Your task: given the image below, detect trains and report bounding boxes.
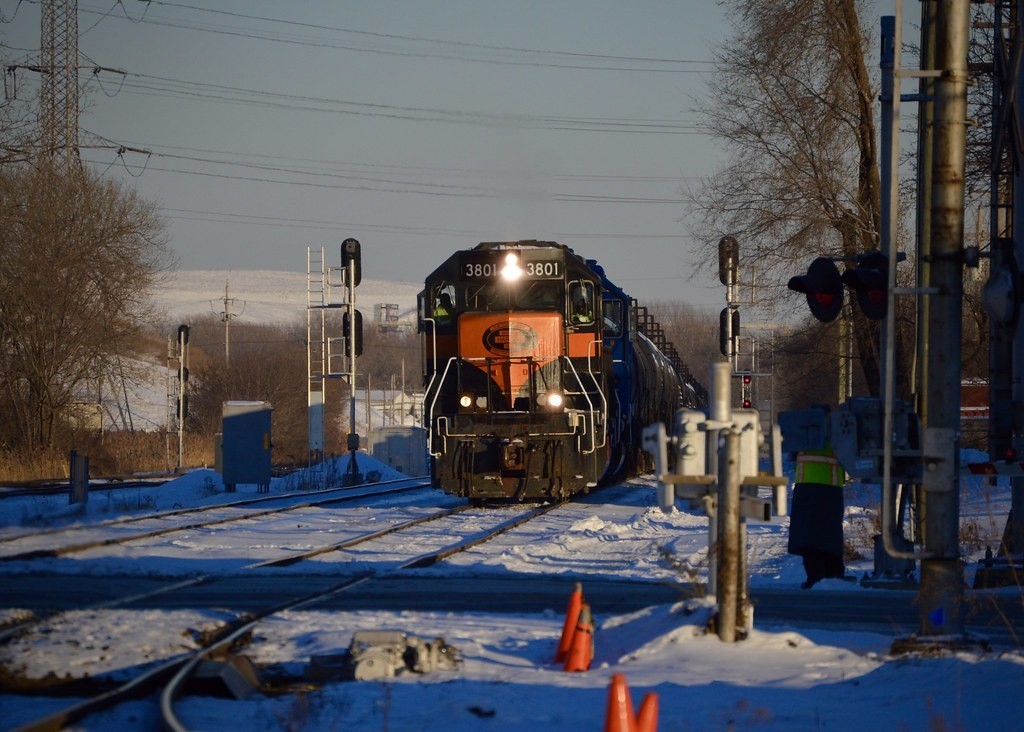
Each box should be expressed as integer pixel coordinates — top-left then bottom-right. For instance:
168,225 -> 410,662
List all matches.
417,239 -> 710,508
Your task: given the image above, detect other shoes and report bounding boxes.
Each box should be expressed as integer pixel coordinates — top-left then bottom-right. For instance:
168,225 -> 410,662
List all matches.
801,580 -> 816,589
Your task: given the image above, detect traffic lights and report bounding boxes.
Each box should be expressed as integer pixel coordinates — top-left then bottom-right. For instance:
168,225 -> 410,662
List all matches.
788,257 -> 844,323
842,250 -> 888,321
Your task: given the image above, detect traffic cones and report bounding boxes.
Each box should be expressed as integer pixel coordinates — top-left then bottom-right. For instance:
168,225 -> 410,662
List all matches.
562,603 -> 595,674
636,692 -> 659,732
602,672 -> 640,732
551,581 -> 584,665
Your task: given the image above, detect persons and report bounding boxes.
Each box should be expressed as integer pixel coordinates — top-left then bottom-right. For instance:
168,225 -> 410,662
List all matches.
434,294 -> 454,316
572,299 -> 592,322
790,404 -> 844,589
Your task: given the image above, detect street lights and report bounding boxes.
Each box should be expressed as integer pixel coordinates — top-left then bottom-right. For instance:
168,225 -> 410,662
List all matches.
744,368 -> 751,383
744,399 -> 751,408
